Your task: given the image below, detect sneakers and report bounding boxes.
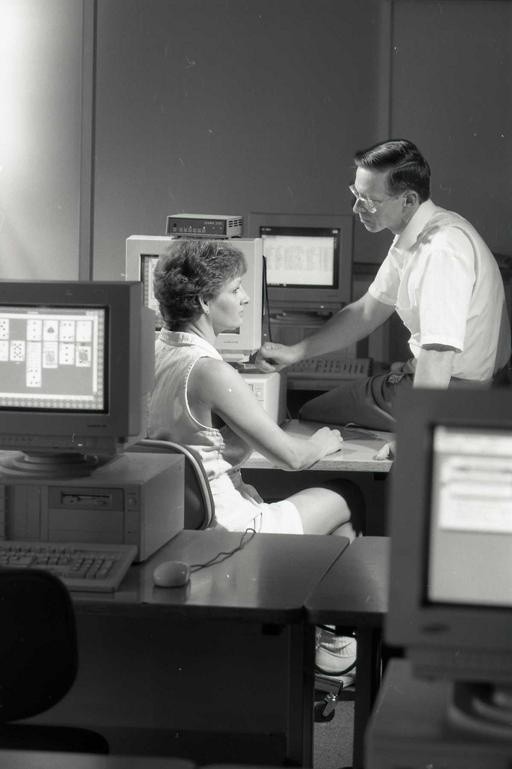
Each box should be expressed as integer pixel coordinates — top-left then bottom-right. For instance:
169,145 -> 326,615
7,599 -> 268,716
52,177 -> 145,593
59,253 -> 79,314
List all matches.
312,623 -> 356,675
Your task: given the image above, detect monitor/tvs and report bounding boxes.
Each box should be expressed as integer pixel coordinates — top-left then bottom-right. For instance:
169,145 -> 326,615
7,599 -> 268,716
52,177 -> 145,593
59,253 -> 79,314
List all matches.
246,212 -> 356,324
125,235 -> 265,362
383,387 -> 512,739
0,280 -> 156,477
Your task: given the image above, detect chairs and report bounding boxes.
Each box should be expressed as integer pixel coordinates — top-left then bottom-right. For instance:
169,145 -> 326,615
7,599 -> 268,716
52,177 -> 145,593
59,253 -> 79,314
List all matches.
0,568 -> 108,754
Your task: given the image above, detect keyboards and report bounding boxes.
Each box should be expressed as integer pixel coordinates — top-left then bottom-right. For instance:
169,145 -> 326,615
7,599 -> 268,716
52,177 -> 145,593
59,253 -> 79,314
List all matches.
0,540 -> 139,594
284,358 -> 373,380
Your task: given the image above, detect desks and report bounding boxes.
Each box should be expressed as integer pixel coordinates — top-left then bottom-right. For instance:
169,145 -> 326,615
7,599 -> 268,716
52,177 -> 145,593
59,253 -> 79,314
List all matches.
233,420 -> 391,538
306,535 -> 391,766
72,529 -> 352,767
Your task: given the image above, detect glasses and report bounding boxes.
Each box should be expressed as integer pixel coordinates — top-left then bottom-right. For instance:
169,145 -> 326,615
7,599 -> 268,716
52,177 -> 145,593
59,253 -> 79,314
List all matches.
349,180 -> 402,213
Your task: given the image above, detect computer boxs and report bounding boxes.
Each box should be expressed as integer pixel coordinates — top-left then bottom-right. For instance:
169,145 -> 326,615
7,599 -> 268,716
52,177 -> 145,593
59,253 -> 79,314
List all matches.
0,450 -> 185,563
234,362 -> 287,429
263,323 -> 368,359
361,656 -> 512,769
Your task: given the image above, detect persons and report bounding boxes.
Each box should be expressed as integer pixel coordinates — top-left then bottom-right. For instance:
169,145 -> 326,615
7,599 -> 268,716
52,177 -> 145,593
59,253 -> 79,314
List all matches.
137,236 -> 362,678
251,136 -> 512,534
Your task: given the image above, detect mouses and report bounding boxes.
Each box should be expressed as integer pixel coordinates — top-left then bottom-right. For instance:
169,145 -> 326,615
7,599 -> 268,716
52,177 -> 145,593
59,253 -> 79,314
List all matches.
390,361 -> 405,371
153,560 -> 190,586
